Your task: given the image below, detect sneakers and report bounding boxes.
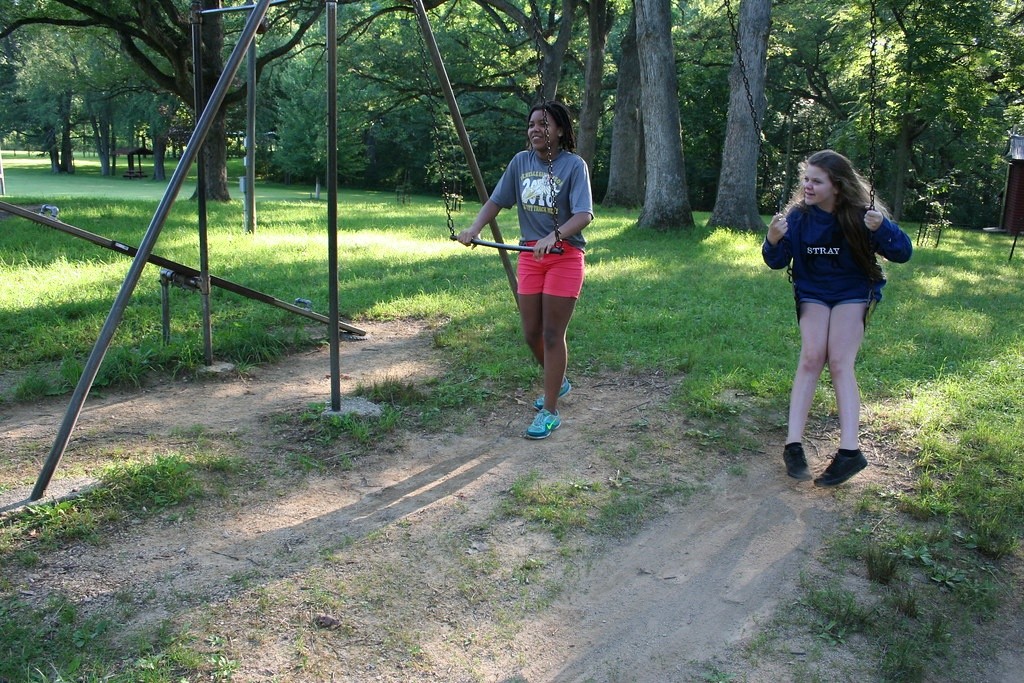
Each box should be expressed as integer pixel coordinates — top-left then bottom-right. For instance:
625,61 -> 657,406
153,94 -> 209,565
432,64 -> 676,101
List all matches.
524,407 -> 561,439
783,445 -> 812,481
533,375 -> 571,411
814,449 -> 867,488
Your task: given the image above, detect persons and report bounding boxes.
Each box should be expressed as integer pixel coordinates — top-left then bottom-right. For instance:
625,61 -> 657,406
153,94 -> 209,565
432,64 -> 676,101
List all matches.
456,100 -> 594,439
762,149 -> 914,486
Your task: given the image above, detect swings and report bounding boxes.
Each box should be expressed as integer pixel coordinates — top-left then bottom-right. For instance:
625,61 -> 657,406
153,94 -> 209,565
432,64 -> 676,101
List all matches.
416,0 -> 564,255
725,2 -> 882,333
